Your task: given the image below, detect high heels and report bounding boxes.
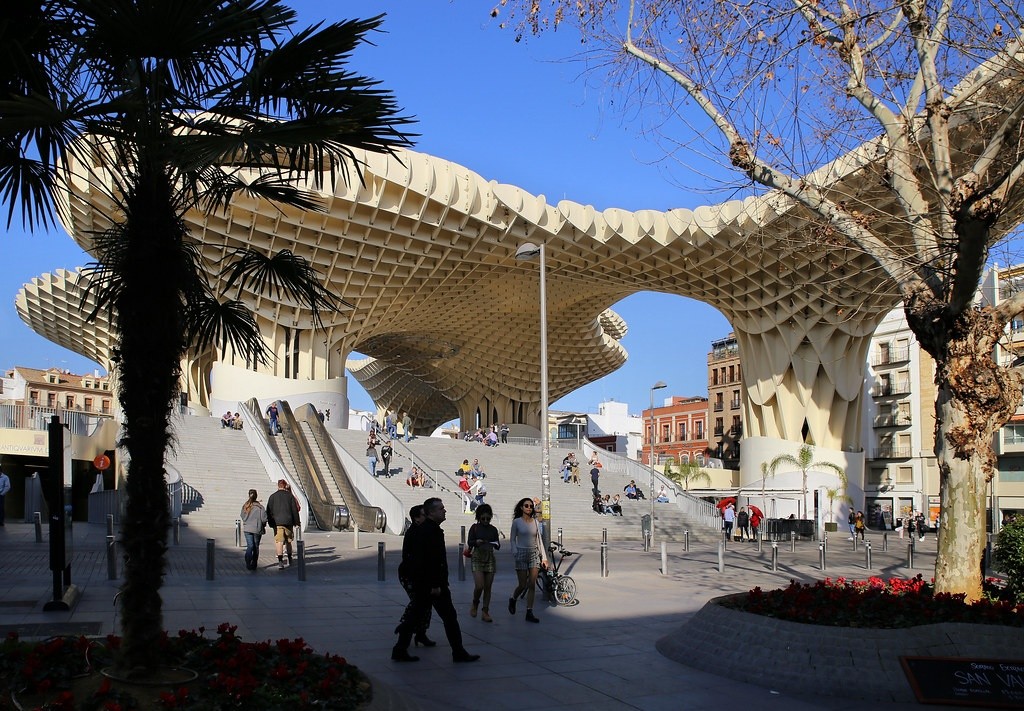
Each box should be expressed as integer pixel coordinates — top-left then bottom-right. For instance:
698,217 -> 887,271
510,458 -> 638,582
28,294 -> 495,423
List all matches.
414,635 -> 436,647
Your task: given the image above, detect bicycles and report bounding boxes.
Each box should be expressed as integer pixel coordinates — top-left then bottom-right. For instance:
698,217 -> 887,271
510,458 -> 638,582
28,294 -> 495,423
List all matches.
520,540 -> 578,606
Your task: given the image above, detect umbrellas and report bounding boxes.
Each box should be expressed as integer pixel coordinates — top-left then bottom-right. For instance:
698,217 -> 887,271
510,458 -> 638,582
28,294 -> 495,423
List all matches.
716,497 -> 736,509
746,505 -> 764,518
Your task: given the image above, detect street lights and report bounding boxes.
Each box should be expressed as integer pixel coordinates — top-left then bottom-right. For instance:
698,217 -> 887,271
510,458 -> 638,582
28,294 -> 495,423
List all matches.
515,242 -> 553,572
650,382 -> 668,548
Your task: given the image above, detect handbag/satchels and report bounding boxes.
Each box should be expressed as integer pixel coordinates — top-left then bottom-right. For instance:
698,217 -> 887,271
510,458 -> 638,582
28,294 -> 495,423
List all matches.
536,532 -> 547,561
595,462 -> 602,467
464,549 -> 473,558
383,451 -> 390,459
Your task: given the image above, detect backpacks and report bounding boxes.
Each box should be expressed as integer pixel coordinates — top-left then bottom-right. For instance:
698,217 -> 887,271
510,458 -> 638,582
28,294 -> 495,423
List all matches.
478,484 -> 487,496
455,468 -> 464,476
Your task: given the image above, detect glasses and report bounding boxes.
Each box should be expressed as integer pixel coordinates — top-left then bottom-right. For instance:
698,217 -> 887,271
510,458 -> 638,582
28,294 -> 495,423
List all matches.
524,504 -> 534,508
481,517 -> 491,521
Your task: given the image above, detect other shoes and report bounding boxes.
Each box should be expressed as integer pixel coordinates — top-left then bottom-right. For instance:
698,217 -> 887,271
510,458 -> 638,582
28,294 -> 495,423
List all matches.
525,609 -> 540,623
481,608 -> 493,622
278,561 -> 285,570
471,599 -> 480,617
288,557 -> 293,564
391,646 -> 421,662
452,649 -> 480,663
508,597 -> 517,615
246,561 -> 258,571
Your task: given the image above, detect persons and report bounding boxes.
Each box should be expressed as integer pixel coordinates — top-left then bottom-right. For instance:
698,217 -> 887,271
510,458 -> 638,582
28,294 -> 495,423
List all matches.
721,501 -> 760,542
390,497 -> 482,663
460,458 -> 484,480
276,485 -> 301,558
394,504 -> 438,648
221,411 -> 243,430
468,503 -> 501,622
847,507 -> 865,543
386,410 -> 412,443
407,467 -> 425,490
380,441 -> 393,478
559,450 -> 669,516
0,464 -> 10,526
240,489 -> 268,570
266,402 -> 279,436
934,512 -> 940,540
458,473 -> 487,513
366,442 -> 380,476
267,480 -> 300,569
508,498 -> 548,623
534,497 -> 543,535
318,409 -> 325,424
464,422 -> 510,447
367,430 -> 376,445
906,510 -> 926,542
